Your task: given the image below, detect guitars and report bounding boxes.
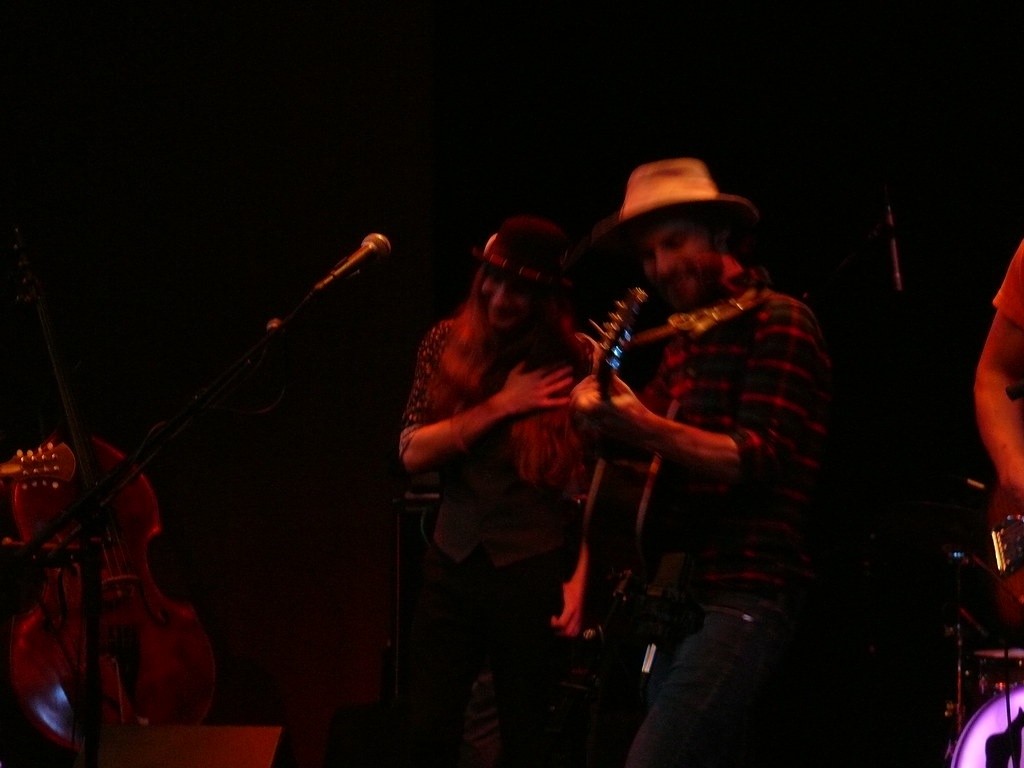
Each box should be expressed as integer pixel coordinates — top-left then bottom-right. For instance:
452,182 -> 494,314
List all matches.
535,279 -> 659,652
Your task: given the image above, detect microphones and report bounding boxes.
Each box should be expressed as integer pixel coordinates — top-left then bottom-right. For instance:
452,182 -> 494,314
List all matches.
889,206 -> 902,293
313,233 -> 392,291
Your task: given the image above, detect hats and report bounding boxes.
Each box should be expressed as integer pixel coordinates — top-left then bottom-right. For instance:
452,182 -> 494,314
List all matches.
593,158 -> 763,243
473,215 -> 577,291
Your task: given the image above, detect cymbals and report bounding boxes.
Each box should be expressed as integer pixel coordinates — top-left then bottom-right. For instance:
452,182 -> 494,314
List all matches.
974,644 -> 1024,660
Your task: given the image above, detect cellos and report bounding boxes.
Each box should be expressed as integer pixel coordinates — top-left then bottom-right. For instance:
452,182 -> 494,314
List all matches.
0,207 -> 227,761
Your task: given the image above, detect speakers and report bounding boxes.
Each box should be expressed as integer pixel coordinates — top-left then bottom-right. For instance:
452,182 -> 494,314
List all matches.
393,503 -> 503,768
74,723 -> 298,768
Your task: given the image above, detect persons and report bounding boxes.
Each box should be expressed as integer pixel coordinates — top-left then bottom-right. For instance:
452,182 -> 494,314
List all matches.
571,158 -> 833,768
972,238 -> 1024,627
396,217 -> 607,768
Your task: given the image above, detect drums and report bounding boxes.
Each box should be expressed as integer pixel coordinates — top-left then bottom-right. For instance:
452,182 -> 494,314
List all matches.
936,669 -> 1024,768
